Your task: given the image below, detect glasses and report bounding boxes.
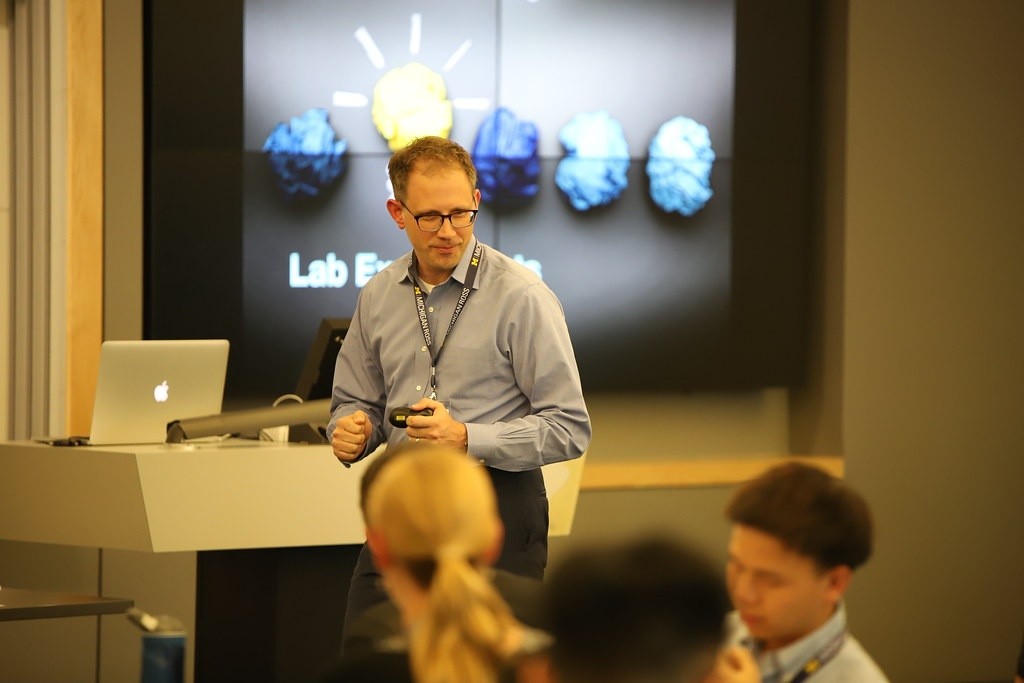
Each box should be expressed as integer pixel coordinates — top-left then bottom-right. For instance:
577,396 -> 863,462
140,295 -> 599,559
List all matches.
400,196 -> 478,234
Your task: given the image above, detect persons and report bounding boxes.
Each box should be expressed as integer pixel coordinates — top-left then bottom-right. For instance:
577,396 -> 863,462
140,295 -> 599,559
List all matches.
540,531 -> 737,683
314,440 -> 560,683
715,462 -> 892,683
324,136 -> 593,657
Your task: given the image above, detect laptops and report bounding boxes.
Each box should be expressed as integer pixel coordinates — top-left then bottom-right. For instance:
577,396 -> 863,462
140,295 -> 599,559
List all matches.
34,339 -> 231,446
